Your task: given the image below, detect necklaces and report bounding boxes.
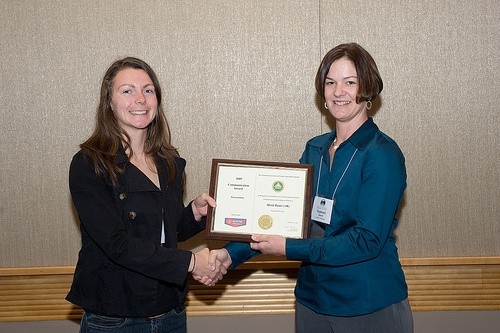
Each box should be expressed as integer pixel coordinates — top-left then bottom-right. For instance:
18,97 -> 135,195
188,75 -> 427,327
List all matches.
331,136 -> 350,150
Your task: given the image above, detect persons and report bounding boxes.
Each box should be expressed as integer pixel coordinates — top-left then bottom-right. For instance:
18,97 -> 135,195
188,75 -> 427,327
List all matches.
65,56 -> 228,333
192,41 -> 414,333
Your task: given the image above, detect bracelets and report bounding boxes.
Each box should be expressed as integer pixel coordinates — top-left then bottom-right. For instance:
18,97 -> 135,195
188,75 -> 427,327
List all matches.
190,251 -> 198,273
